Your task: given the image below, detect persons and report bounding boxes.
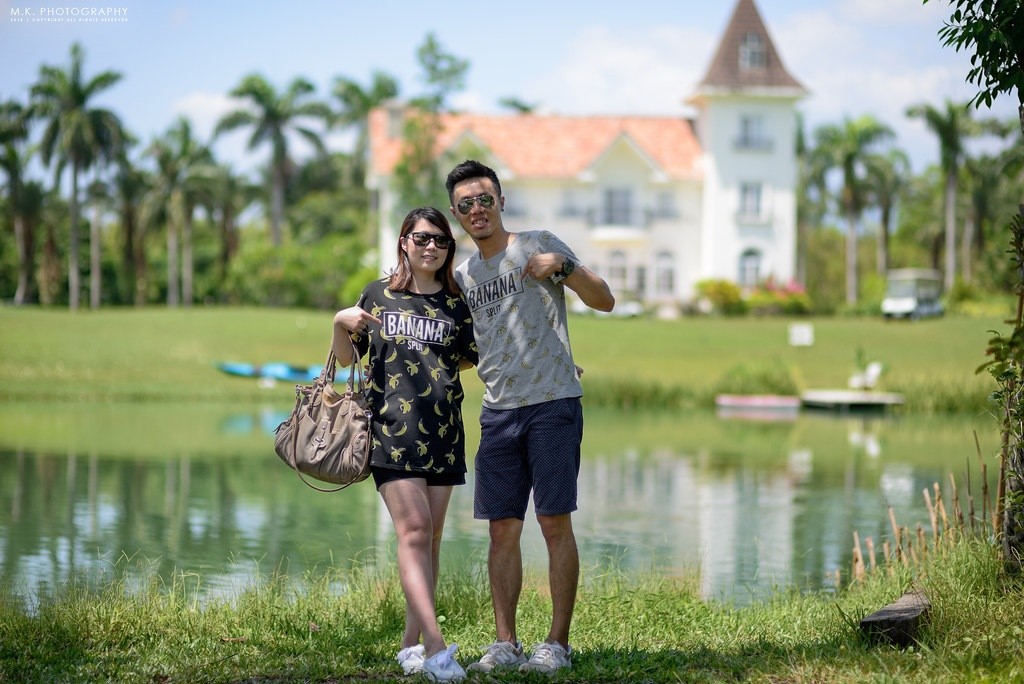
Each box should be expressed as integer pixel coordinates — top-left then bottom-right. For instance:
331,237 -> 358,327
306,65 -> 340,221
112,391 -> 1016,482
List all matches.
332,207 -> 583,684
364,160 -> 615,672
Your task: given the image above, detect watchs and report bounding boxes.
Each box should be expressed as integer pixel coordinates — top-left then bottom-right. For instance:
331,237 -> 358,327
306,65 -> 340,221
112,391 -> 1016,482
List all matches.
555,256 -> 575,280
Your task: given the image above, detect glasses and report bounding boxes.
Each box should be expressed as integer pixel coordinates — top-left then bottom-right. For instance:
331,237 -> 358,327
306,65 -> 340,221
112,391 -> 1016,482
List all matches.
457,194 -> 496,215
406,231 -> 452,249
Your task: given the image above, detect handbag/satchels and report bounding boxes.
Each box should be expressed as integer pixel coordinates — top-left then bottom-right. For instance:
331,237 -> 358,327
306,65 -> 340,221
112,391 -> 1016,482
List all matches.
272,341 -> 372,492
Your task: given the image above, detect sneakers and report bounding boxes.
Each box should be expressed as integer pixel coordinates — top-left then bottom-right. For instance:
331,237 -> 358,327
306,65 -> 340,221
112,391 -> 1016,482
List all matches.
397,643 -> 424,675
423,643 -> 466,683
466,641 -> 528,674
519,638 -> 572,674
